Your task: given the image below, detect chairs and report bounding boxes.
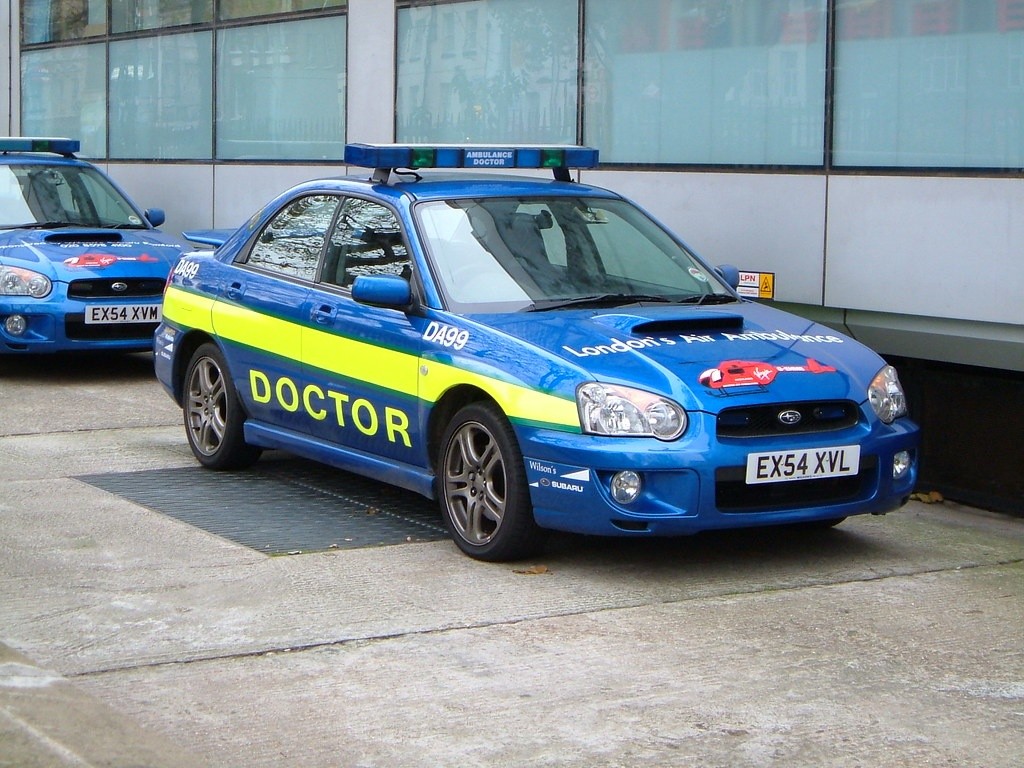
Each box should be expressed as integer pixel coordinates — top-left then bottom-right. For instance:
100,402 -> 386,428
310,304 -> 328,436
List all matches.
28,173 -> 69,224
497,213 -> 560,294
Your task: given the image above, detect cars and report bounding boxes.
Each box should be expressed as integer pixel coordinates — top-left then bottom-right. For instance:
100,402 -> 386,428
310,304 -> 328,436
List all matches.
0,136 -> 186,361
151,140 -> 921,562
